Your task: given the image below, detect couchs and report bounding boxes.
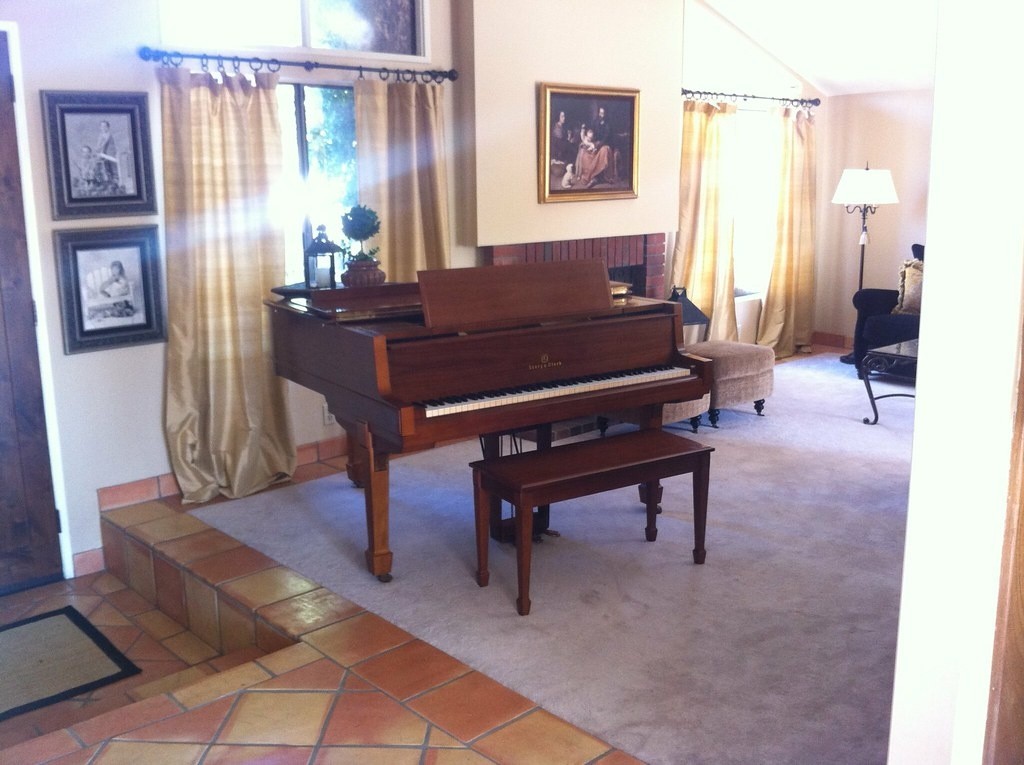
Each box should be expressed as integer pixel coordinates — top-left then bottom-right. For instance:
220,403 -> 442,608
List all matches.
852,244 -> 924,366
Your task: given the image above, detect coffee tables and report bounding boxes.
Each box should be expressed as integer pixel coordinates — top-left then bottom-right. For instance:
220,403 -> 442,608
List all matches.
860,336 -> 920,426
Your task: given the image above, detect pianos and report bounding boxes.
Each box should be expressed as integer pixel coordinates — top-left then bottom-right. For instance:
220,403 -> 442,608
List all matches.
263,257 -> 715,582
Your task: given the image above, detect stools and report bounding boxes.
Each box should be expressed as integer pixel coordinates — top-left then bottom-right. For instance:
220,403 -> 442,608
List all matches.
598,340 -> 779,435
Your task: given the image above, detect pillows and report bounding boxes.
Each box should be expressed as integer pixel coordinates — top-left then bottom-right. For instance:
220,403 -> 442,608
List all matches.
893,258 -> 924,315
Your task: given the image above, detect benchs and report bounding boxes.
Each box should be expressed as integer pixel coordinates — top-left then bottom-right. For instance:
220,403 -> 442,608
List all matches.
470,431 -> 714,617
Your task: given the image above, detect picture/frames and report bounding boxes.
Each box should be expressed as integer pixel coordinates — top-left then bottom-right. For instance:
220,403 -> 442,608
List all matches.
340,205 -> 387,288
536,82 -> 643,204
52,224 -> 169,356
38,90 -> 160,220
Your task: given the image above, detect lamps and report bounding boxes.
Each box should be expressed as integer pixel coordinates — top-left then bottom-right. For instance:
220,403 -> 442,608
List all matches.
831,159 -> 899,363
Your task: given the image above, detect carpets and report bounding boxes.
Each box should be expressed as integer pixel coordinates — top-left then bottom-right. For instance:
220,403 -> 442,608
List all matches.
0,605 -> 143,725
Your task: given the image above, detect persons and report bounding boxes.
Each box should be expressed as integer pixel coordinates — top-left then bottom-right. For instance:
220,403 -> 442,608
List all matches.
552,106 -> 615,190
81,121 -> 119,188
99,261 -> 134,317
